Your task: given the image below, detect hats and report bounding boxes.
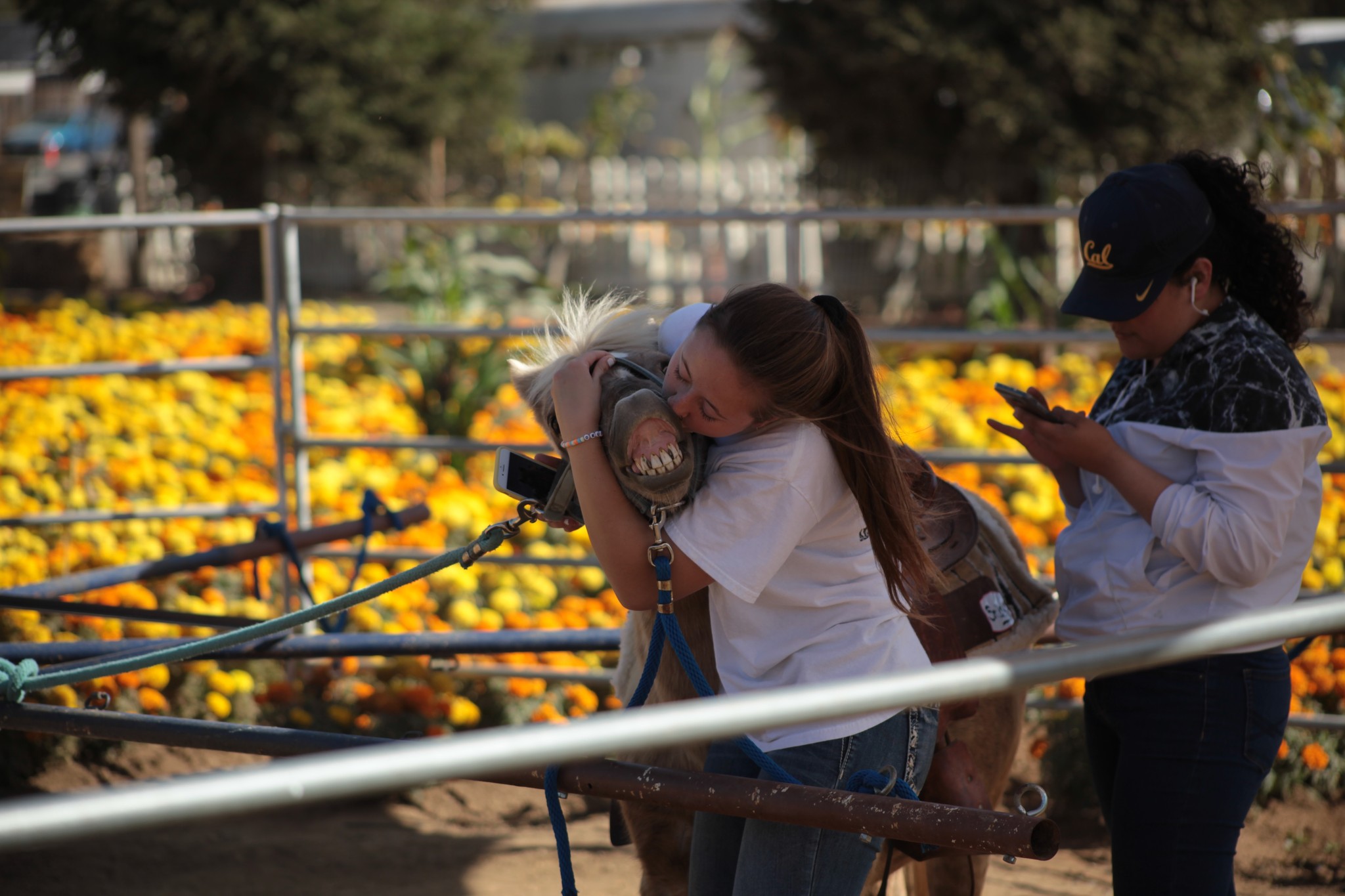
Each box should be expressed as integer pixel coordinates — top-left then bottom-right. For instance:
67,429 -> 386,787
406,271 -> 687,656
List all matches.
1056,167 -> 1216,320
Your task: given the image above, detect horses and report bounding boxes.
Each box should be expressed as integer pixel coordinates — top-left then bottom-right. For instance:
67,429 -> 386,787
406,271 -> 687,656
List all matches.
507,283 -> 1055,895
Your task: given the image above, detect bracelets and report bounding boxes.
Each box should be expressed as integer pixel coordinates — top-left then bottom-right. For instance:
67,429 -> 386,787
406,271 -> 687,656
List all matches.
561,431 -> 603,448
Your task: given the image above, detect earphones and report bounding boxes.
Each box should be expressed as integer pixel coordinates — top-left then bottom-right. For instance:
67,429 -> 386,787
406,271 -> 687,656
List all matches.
1190,277 -> 1198,305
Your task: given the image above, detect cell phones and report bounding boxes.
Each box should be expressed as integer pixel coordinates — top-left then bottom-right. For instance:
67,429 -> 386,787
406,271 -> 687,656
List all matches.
994,382 -> 1063,424
493,446 -> 570,518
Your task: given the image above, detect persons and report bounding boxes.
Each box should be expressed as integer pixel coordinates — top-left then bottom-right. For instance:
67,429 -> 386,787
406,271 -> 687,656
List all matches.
533,283 -> 941,896
985,146 -> 1331,896
